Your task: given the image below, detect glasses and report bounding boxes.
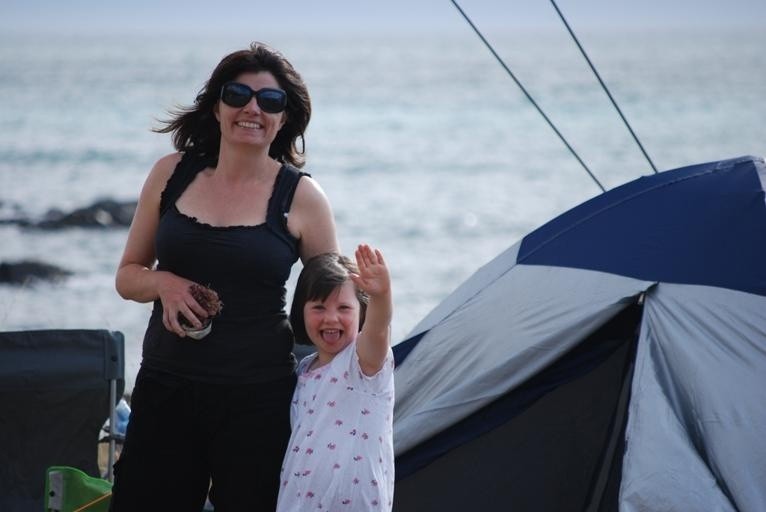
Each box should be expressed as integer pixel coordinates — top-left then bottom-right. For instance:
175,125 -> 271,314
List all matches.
220,80 -> 289,114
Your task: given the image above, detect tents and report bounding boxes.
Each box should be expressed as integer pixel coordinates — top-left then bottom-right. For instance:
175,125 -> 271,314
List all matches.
395,155 -> 766,512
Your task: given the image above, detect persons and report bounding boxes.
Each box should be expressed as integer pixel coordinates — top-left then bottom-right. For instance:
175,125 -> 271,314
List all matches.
108,39 -> 342,512
275,244 -> 395,512
